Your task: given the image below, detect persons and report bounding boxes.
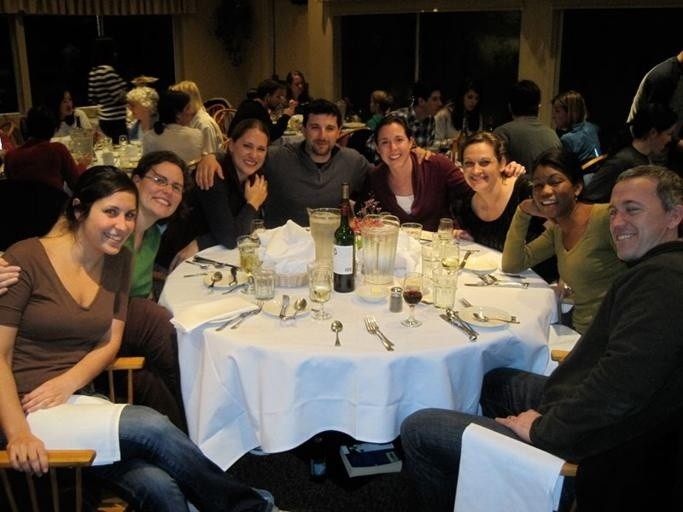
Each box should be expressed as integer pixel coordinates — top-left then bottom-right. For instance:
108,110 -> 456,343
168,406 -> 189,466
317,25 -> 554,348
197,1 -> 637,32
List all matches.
157,114 -> 270,273
0,165 -> 271,508
228,68 -> 312,143
502,146 -> 630,336
351,115 -> 527,248
0,149 -> 191,297
400,164 -> 683,508
452,131 -> 559,285
195,98 -> 436,227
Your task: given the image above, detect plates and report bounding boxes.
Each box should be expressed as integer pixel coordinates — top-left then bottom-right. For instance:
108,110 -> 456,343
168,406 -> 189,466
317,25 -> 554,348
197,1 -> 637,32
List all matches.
208,312 -> 240,324
456,254 -> 498,272
342,123 -> 365,128
201,269 -> 248,288
459,304 -> 512,329
262,297 -> 310,319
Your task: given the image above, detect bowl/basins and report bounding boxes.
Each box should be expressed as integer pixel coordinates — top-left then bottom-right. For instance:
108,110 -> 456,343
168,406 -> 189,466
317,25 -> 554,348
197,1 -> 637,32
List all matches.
352,285 -> 387,303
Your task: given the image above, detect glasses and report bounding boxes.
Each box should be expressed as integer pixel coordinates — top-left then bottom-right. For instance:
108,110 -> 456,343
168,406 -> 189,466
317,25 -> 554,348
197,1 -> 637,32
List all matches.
143,175 -> 185,195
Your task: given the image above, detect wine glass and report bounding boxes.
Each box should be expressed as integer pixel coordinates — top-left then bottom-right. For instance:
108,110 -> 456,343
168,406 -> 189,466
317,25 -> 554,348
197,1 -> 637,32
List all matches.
306,263 -> 337,320
401,271 -> 426,329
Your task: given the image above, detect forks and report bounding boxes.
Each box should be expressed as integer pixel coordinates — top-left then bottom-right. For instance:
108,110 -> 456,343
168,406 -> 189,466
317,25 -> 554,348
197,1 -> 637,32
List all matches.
459,298 -> 471,307
215,300 -> 267,333
363,313 -> 395,352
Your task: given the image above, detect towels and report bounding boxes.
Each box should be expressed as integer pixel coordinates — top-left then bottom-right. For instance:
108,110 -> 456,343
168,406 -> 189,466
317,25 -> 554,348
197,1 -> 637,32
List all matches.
452,423 -> 568,512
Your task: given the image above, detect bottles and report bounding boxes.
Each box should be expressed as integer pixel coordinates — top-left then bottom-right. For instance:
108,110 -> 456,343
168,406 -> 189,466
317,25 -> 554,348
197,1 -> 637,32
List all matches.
339,182 -> 355,230
389,287 -> 403,313
334,205 -> 355,293
451,138 -> 459,166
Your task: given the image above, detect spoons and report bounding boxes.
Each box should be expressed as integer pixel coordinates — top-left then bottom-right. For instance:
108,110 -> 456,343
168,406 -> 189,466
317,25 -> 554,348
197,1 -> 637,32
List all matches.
330,319 -> 344,348
464,273 -> 530,289
208,269 -> 222,288
183,257 -> 235,269
469,309 -> 523,325
288,296 -> 306,321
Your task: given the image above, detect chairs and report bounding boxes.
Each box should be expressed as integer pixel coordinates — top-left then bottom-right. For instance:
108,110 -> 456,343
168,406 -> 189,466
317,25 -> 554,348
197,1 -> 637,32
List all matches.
0,355 -> 145,512
455,393 -> 683,512
580,153 -> 609,173
336,127 -> 372,152
203,98 -> 238,133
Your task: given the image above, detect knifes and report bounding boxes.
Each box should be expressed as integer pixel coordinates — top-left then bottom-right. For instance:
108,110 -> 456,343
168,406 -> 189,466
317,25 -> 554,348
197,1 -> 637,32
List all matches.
221,282 -> 248,295
438,308 -> 477,340
227,266 -> 237,286
460,250 -> 473,269
279,294 -> 291,317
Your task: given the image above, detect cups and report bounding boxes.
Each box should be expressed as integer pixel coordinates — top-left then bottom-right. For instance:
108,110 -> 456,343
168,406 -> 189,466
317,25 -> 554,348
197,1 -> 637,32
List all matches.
359,212 -> 400,284
309,207 -> 341,264
235,234 -> 261,269
68,127 -> 93,162
400,217 -> 461,313
351,116 -> 358,123
101,135 -> 138,168
250,218 -> 264,236
252,262 -> 276,300
